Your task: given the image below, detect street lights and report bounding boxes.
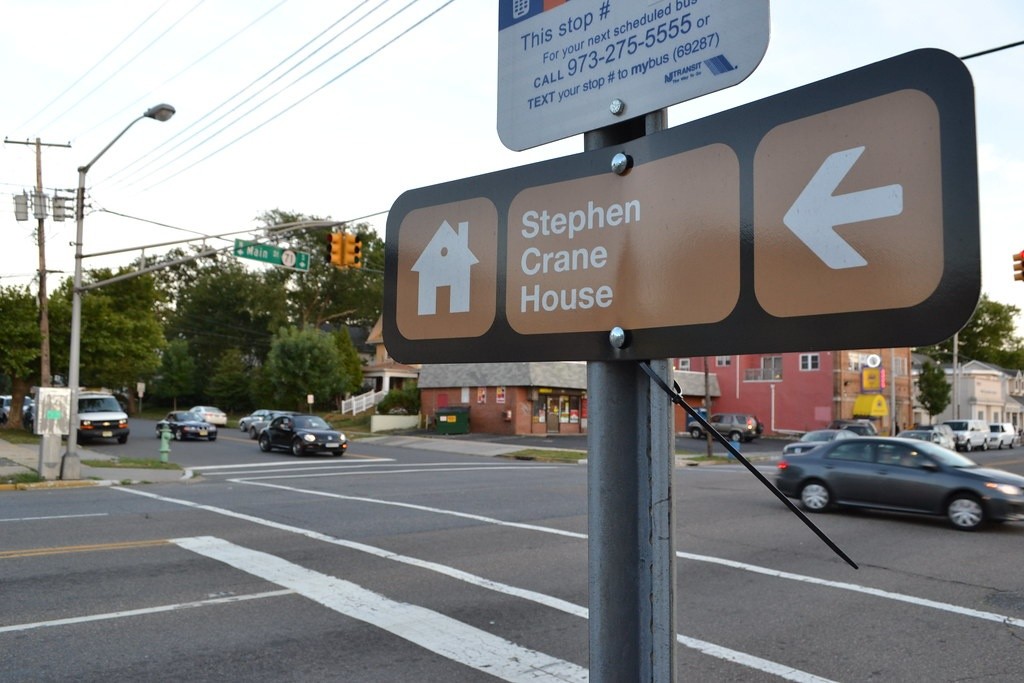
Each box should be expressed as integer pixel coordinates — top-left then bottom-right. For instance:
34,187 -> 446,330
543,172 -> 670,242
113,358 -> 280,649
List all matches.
57,104 -> 177,482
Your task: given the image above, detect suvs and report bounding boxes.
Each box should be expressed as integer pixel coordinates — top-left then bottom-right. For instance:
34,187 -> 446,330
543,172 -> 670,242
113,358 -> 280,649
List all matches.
689,411 -> 764,442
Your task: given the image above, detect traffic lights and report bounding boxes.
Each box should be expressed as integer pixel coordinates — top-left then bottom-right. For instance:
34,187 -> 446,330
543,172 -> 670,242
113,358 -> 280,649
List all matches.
324,232 -> 364,267
1013,249 -> 1024,283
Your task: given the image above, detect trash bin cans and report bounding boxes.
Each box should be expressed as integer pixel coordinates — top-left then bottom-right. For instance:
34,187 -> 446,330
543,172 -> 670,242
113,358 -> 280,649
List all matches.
728,441 -> 741,460
434,405 -> 472,435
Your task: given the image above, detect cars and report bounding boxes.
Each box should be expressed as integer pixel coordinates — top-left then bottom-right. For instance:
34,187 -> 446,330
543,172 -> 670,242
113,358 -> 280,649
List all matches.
774,437 -> 1024,533
797,416 -> 1018,453
71,390 -> 130,444
188,405 -> 228,426
238,407 -> 348,458
155,410 -> 218,442
0,394 -> 33,424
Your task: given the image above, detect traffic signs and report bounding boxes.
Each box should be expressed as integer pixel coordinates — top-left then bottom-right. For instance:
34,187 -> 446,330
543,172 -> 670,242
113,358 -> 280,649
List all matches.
383,46 -> 984,366
